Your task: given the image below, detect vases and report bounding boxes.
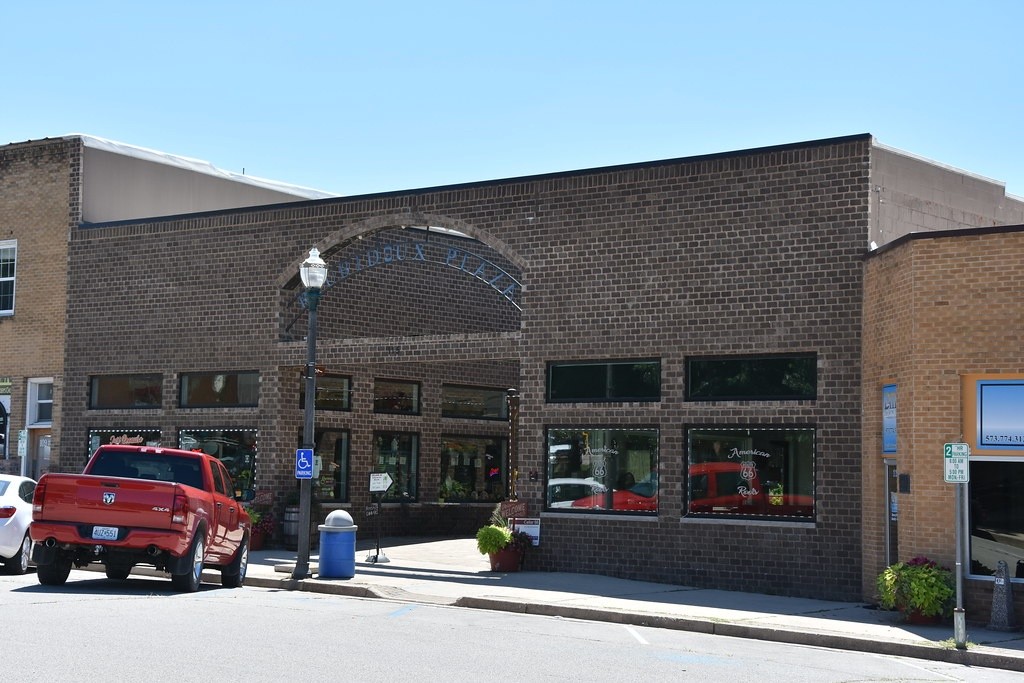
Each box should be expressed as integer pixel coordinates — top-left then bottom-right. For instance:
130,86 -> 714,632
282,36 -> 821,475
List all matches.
896,596 -> 939,624
248,531 -> 267,551
488,547 -> 523,572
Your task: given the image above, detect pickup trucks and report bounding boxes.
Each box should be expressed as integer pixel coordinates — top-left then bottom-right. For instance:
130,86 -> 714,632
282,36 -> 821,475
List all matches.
29,444 -> 257,594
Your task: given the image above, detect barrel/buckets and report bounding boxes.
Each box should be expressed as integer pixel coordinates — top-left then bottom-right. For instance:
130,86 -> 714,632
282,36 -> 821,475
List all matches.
283,505 -> 316,550
317,509 -> 357,581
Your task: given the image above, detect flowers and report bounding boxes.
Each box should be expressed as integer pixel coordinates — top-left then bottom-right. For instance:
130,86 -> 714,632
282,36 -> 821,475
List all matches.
874,556 -> 956,618
476,524 -> 532,555
244,508 -> 276,534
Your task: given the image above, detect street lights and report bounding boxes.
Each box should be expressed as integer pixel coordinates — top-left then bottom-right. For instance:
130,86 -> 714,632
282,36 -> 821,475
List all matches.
291,241 -> 330,581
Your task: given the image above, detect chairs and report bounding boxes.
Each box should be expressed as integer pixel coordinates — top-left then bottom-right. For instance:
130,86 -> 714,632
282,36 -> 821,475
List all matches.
98,454 -> 158,480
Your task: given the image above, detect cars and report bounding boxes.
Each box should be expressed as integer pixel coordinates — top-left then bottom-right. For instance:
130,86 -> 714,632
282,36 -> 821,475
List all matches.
0,474 -> 39,576
547,442 -> 814,516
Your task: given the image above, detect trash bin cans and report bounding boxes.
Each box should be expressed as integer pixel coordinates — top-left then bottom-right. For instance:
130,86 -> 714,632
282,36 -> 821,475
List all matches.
318,509 -> 358,578
